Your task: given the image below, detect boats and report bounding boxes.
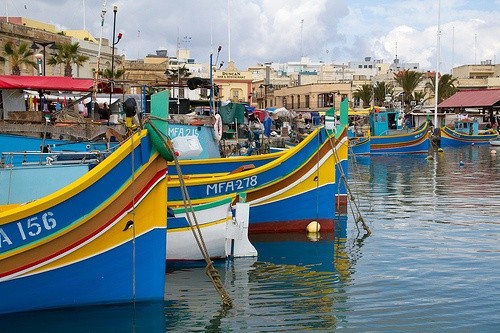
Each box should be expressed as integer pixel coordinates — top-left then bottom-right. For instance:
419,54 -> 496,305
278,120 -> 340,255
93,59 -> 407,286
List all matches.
0,89 -> 500,314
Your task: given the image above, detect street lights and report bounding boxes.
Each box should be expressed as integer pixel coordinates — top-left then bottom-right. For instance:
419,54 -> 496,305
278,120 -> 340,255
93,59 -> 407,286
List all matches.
106,6 -> 123,127
29,39 -> 55,92
209,45 -> 225,116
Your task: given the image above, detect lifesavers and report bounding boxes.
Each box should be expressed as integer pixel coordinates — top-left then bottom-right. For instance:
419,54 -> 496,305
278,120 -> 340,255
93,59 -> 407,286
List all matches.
213,114 -> 223,142
